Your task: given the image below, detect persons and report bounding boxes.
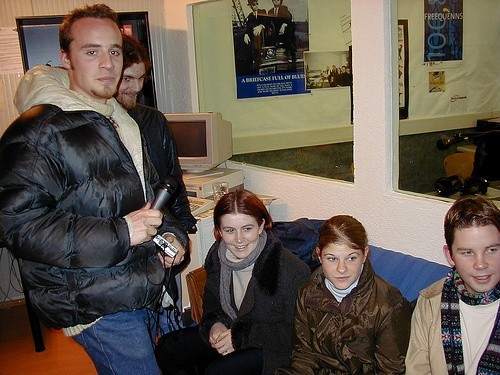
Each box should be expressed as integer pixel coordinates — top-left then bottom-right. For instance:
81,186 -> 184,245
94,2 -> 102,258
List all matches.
404,195 -> 500,375
115,31 -> 199,335
1,4 -> 192,375
243,0 -> 297,67
276,215 -> 412,375
156,189 -> 310,375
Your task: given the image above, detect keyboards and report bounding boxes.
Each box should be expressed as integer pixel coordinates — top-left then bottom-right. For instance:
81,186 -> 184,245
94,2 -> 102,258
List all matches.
187,196 -> 215,217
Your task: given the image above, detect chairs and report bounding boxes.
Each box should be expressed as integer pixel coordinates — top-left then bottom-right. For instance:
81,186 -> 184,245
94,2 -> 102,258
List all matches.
443,153 -> 475,186
456,129 -> 500,182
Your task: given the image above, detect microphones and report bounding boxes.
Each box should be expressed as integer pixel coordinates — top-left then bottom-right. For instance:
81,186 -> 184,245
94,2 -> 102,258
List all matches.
150,175 -> 179,212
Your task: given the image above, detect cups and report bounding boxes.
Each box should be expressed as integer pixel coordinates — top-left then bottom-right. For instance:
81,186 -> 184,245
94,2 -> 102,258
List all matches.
212,181 -> 229,204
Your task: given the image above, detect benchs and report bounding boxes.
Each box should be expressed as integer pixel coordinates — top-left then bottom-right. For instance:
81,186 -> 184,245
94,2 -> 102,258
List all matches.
186,244 -> 451,327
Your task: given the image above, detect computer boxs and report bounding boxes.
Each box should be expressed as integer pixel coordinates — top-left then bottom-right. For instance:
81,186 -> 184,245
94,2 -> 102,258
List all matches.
181,168 -> 244,200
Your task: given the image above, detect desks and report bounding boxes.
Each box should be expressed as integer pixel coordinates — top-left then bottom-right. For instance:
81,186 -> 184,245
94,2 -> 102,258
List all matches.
175,195 -> 280,314
0,238 -> 47,352
424,180 -> 500,211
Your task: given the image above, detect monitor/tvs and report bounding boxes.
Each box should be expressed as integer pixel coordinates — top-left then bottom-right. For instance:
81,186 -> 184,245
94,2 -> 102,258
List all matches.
161,112 -> 232,180
